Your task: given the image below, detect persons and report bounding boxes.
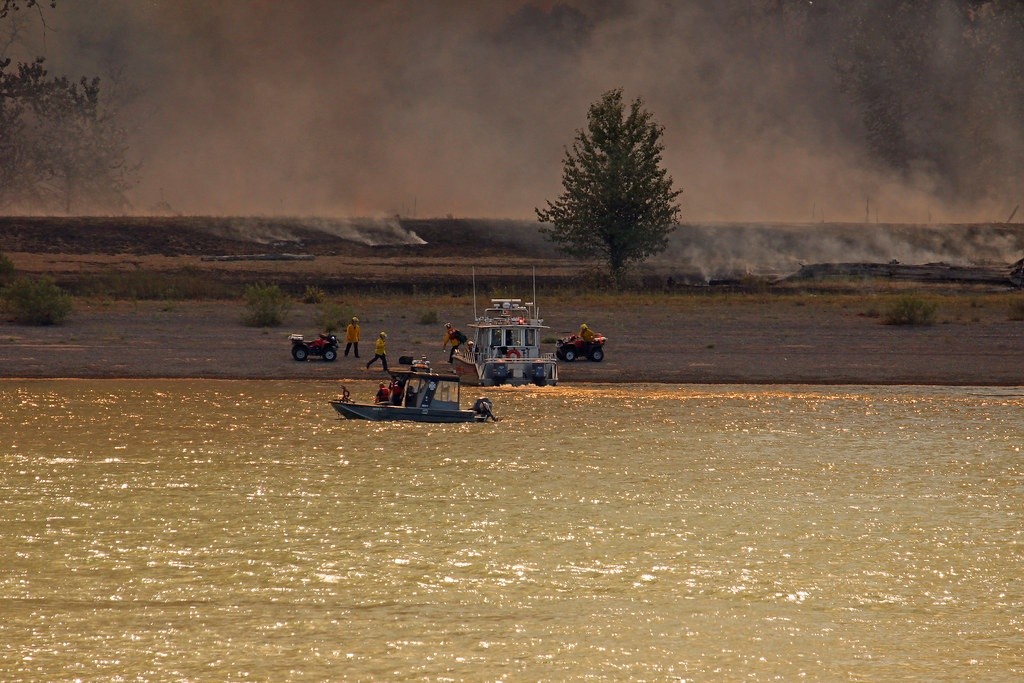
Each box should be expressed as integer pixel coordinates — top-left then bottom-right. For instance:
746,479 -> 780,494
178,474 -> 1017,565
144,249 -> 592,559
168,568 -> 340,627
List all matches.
365,332 -> 390,374
578,324 -> 596,342
345,317 -> 362,358
443,323 -> 461,363
375,375 -> 414,404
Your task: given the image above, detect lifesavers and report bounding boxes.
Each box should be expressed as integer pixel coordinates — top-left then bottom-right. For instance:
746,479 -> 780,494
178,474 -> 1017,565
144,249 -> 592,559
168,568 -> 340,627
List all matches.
506,349 -> 520,362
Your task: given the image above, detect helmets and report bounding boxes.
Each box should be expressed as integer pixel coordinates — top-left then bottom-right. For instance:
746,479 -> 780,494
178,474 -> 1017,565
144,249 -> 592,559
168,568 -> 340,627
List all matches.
467,341 -> 473,346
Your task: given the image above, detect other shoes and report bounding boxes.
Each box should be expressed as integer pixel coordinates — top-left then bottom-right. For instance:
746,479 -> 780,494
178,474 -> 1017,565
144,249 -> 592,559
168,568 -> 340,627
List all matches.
355,355 -> 360,358
447,360 -> 453,363
345,352 -> 348,356
383,368 -> 389,371
367,362 -> 370,369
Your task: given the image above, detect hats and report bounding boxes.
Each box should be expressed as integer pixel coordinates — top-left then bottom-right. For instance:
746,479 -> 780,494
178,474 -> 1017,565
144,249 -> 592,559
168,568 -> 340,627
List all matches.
444,323 -> 451,328
580,324 -> 588,329
352,317 -> 359,322
380,332 -> 387,338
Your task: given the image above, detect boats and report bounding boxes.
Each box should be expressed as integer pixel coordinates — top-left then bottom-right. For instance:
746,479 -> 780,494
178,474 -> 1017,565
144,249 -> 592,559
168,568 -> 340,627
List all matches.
451,267 -> 558,388
328,354 -> 498,423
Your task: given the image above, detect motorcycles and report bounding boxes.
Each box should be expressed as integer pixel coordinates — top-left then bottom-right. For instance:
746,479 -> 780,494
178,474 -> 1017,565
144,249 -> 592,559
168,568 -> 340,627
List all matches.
555,333 -> 608,362
287,331 -> 340,361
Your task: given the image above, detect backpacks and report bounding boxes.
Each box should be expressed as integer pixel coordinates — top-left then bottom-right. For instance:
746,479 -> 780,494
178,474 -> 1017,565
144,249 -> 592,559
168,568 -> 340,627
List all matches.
454,331 -> 467,343
